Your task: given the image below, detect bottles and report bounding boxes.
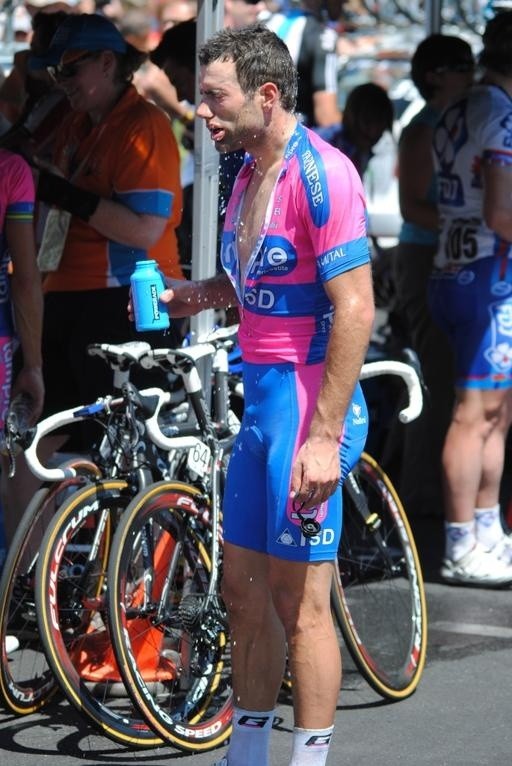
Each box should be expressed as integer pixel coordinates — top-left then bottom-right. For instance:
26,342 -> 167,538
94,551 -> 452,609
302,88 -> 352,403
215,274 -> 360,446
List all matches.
131,257 -> 172,331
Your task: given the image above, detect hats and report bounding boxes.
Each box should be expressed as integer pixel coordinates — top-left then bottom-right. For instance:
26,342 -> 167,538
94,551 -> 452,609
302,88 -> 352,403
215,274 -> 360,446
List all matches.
40,13 -> 127,66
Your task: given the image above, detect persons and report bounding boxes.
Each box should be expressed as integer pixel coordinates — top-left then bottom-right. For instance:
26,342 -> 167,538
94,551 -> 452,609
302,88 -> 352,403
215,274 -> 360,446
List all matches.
0,0 -> 511,766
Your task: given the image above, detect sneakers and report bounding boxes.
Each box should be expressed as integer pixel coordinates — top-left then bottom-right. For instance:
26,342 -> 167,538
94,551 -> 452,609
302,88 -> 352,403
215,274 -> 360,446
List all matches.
438,534 -> 512,586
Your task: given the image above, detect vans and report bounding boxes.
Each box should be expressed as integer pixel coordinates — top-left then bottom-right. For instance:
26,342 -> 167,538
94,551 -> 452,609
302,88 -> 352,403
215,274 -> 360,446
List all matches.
335,46 -> 424,239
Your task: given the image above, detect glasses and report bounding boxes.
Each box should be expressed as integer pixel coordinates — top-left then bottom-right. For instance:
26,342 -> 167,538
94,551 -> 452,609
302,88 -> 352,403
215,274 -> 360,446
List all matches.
45,48 -> 106,84
